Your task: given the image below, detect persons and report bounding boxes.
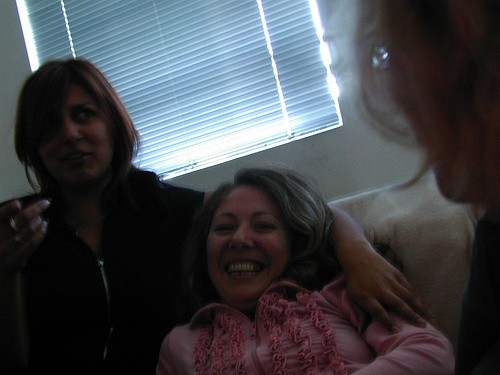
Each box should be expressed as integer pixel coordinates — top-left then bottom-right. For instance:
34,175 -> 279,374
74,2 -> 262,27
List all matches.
325,0 -> 500,375
0,56 -> 437,375
157,167 -> 456,375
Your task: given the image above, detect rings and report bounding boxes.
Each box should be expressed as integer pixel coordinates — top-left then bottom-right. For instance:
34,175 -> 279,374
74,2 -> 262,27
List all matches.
7,216 -> 18,234
